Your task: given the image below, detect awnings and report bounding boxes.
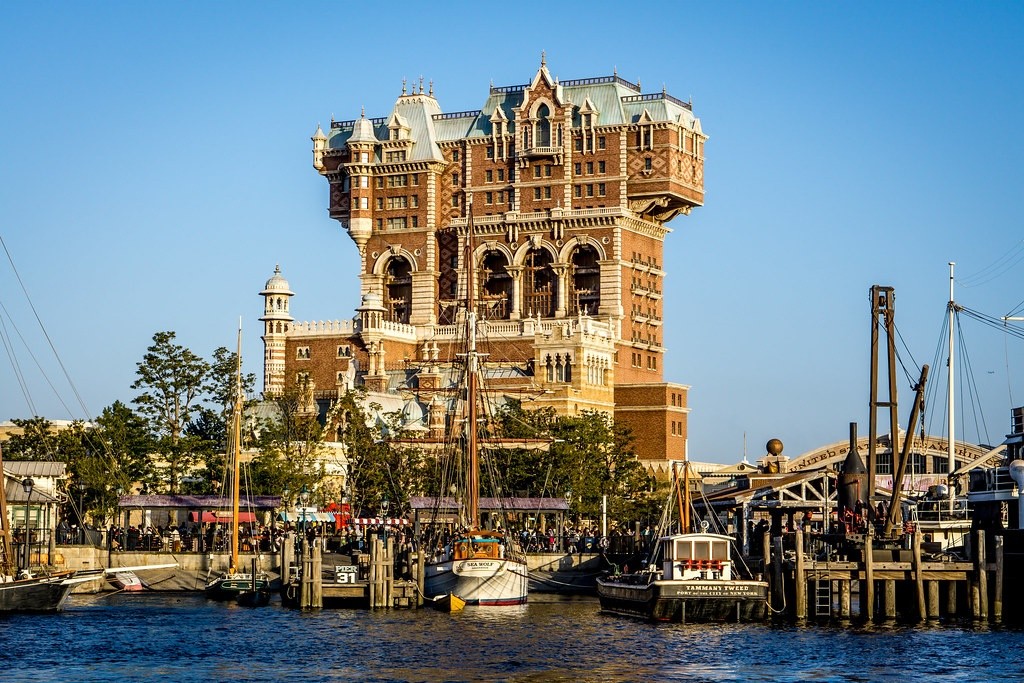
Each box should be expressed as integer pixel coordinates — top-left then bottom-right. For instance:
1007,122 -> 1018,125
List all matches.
275,512 -> 336,523
188,511 -> 257,523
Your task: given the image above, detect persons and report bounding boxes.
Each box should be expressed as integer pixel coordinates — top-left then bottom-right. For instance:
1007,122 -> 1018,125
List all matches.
60,517 -> 651,555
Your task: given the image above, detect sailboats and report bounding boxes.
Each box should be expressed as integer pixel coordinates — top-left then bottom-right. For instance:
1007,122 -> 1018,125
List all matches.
0,445 -> 77,613
424,204 -> 530,610
205,314 -> 271,600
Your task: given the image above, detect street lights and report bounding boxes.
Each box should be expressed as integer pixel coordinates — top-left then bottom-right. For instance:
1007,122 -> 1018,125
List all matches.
450,484 -> 457,530
380,493 -> 389,549
299,483 -> 309,540
340,485 -> 346,538
280,483 -> 290,538
22,475 -> 35,570
296,513 -> 300,567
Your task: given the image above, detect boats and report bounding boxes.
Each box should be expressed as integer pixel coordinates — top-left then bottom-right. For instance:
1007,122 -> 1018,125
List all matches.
115,562 -> 142,591
595,461 -> 769,623
